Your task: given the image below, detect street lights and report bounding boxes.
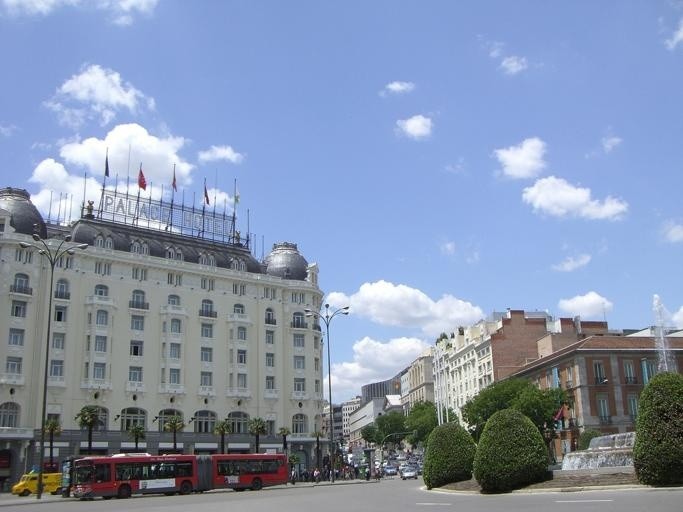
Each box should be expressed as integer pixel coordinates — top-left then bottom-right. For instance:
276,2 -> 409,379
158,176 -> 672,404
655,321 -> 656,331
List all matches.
303,303 -> 351,484
18,232 -> 89,502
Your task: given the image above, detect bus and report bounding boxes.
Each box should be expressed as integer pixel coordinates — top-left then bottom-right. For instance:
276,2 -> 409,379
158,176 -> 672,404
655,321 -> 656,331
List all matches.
70,452 -> 289,502
11,469 -> 62,497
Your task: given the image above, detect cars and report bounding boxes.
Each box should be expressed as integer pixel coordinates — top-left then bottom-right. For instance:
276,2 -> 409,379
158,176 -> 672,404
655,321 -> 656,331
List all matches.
375,448 -> 424,482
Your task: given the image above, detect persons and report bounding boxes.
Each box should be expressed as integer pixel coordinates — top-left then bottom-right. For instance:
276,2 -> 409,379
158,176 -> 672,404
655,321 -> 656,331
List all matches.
81,465 -> 174,483
286,463 -> 385,484
222,462 -> 261,474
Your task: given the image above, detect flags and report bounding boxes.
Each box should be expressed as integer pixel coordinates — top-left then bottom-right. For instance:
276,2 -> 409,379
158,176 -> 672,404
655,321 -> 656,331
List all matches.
104,155 -> 109,177
137,167 -> 147,191
235,188 -> 240,204
172,173 -> 177,193
203,187 -> 210,205
